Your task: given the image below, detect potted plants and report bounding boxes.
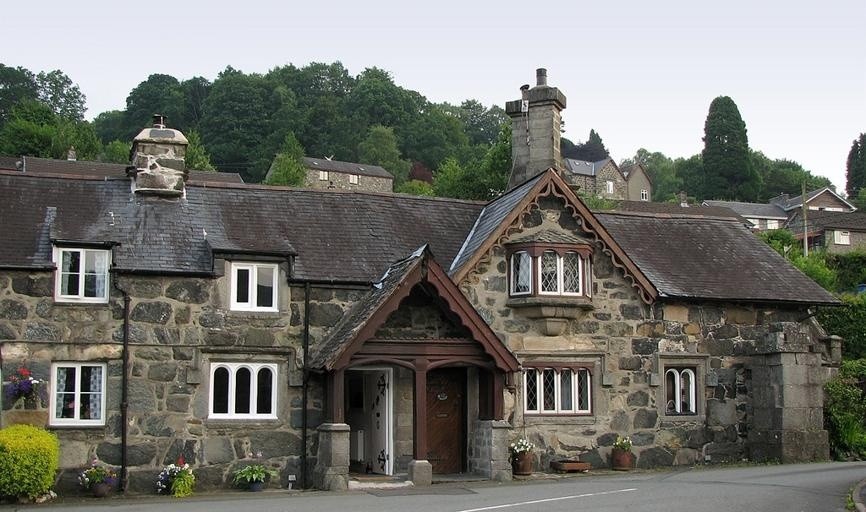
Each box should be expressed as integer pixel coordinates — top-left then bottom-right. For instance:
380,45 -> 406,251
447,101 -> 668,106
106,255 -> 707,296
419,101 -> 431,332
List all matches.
227,460 -> 272,491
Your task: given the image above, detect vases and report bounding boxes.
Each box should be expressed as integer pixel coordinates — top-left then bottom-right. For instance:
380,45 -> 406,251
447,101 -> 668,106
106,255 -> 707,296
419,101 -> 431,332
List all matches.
90,482 -> 110,497
510,448 -> 532,477
610,447 -> 633,473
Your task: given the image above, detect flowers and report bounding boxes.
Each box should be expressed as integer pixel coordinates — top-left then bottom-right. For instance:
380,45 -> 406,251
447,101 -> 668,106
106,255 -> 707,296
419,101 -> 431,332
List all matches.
83,458 -> 119,492
4,366 -> 48,405
509,438 -> 532,455
153,458 -> 198,498
613,435 -> 631,453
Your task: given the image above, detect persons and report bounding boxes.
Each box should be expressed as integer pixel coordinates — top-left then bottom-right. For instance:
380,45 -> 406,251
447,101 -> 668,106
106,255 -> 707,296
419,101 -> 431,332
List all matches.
327,181 -> 335,188
